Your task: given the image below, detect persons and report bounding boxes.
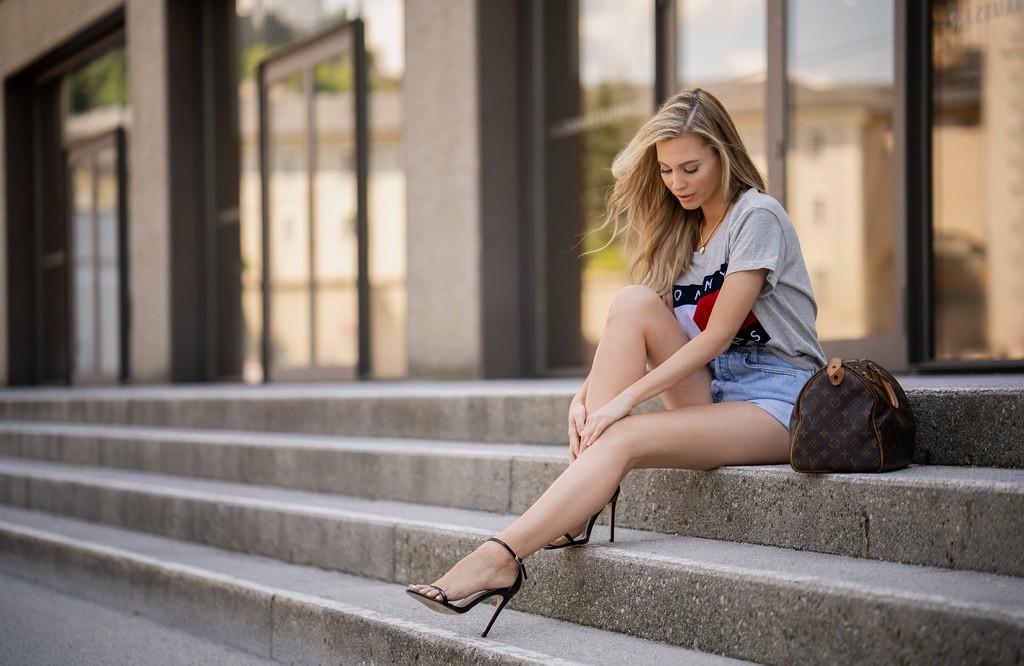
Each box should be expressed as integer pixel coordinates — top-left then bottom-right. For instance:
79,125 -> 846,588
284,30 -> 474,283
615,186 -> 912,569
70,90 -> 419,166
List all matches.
405,86 -> 828,604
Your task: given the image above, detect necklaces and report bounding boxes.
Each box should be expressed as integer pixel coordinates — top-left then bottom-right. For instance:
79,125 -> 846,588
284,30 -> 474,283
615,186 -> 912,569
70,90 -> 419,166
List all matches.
697,199 -> 733,255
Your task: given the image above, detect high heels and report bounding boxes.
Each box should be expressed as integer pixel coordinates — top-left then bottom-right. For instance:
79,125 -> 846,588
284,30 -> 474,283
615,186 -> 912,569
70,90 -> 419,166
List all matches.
408,537 -> 528,638
543,483 -> 621,550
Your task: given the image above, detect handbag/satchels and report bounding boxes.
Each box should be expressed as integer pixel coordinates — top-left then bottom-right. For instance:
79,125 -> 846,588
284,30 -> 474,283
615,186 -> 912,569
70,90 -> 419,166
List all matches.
788,357 -> 913,474
